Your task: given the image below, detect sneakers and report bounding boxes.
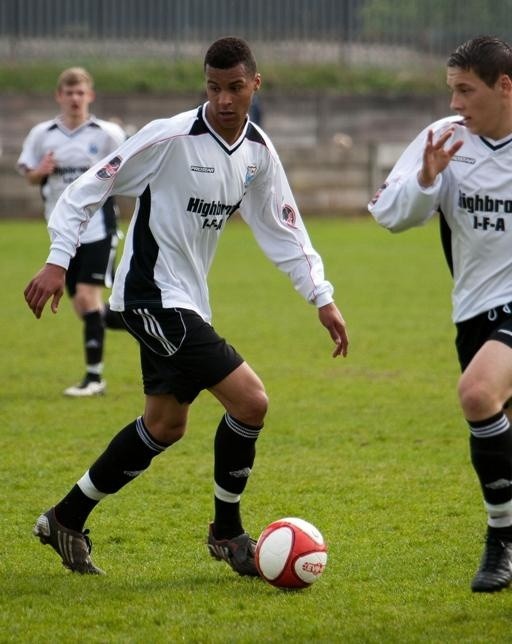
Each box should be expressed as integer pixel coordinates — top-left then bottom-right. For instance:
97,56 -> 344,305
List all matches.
33,504 -> 106,576
207,521 -> 263,577
470,528 -> 512,592
64,372 -> 107,397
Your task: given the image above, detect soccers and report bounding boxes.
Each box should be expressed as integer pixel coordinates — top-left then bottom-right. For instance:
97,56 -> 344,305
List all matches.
255,517 -> 327,588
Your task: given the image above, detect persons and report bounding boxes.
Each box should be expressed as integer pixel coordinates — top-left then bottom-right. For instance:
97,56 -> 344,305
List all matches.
14,65 -> 128,396
365,32 -> 511,592
25,38 -> 350,577
